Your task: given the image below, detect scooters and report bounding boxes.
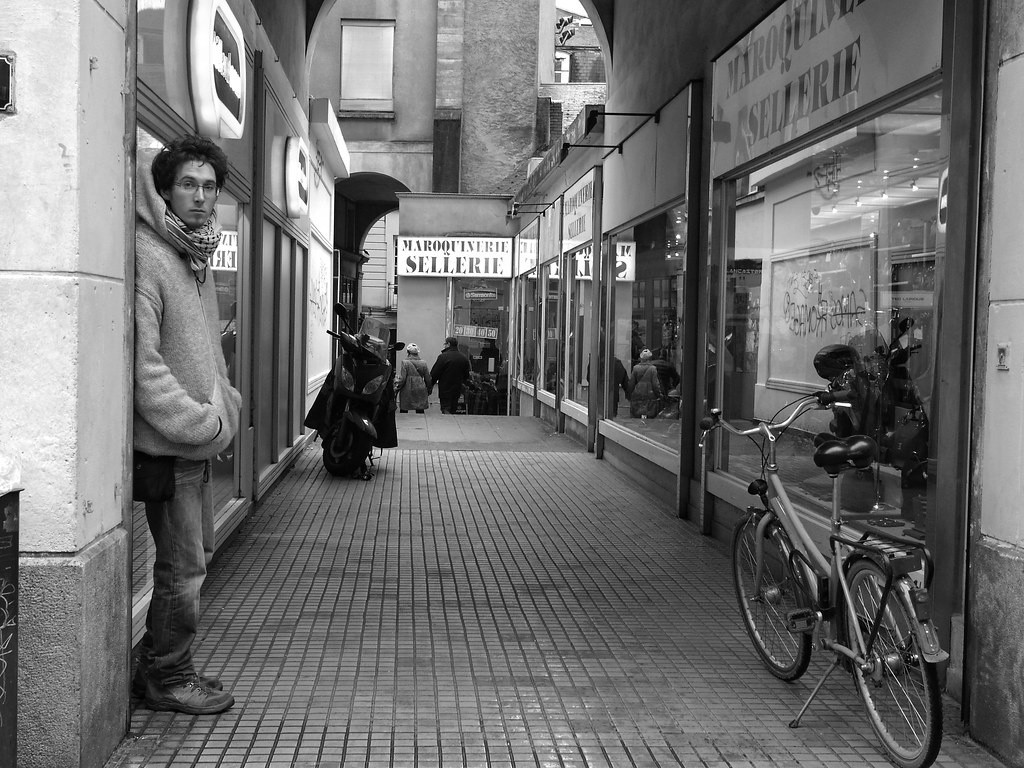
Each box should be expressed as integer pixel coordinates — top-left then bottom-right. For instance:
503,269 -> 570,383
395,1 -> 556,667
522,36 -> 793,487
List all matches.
303,316 -> 405,482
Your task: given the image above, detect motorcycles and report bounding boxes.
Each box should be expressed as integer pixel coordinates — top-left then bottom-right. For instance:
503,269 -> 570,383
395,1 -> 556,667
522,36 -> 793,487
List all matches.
813,315 -> 923,490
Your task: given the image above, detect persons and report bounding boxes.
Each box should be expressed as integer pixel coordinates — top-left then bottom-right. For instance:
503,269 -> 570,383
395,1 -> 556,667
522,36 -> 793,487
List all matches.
628,311 -> 679,419
586,343 -> 630,417
129,132 -> 245,716
394,343 -> 433,414
430,337 -> 470,414
457,337 -> 508,415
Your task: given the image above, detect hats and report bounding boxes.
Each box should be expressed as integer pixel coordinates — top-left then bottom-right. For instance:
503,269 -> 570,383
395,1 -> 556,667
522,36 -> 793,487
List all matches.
640,349 -> 652,358
407,343 -> 420,353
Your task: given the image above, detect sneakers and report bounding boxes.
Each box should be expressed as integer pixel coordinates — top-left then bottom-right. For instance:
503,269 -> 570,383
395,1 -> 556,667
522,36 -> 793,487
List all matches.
131,670 -> 223,696
145,679 -> 235,714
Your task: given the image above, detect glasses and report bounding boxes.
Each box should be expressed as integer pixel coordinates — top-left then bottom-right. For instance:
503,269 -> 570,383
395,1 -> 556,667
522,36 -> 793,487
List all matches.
173,181 -> 220,197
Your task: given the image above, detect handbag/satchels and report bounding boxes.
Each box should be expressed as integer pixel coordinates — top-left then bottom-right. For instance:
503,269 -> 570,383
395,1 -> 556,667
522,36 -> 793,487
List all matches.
839,467 -> 875,514
890,404 -> 930,471
133,450 -> 176,502
897,253 -> 913,290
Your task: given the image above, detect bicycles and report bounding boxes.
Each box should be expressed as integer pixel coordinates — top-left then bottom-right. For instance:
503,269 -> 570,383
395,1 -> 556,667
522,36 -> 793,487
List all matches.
696,383 -> 952,768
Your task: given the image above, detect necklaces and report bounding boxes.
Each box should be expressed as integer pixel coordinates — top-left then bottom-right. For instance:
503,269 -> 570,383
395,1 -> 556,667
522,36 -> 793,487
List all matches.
192,266 -> 207,283
